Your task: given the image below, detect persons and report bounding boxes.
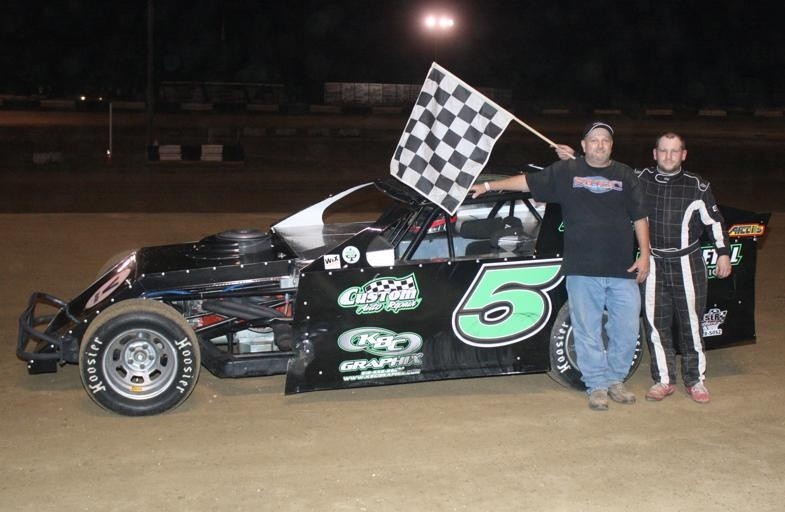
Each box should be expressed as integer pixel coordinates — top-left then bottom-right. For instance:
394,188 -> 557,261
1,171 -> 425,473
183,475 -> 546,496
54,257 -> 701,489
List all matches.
550,130 -> 730,403
470,121 -> 650,410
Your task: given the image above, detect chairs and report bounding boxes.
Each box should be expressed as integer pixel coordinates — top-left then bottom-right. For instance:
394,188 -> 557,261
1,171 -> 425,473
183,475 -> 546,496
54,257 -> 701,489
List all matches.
460,217 -> 523,255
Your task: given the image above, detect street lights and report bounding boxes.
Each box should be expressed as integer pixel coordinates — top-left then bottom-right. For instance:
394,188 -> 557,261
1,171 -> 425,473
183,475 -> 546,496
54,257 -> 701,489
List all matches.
419,10 -> 458,68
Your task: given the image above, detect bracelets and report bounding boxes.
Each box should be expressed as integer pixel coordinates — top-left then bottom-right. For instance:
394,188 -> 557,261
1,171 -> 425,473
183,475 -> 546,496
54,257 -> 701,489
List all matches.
484,182 -> 490,192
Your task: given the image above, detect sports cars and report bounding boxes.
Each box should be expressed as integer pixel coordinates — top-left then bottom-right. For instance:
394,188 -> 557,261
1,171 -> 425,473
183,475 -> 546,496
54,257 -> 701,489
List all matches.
14,174 -> 772,411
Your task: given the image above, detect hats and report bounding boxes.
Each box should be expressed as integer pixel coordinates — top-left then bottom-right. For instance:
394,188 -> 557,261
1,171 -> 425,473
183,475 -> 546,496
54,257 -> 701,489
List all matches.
581,122 -> 614,139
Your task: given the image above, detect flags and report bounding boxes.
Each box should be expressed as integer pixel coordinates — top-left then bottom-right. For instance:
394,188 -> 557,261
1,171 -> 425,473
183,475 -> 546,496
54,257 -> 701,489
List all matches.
389,61 -> 515,215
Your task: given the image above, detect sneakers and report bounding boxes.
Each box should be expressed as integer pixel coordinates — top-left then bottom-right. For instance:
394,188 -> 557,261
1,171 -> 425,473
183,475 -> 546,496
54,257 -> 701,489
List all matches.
589,382 -> 710,410
